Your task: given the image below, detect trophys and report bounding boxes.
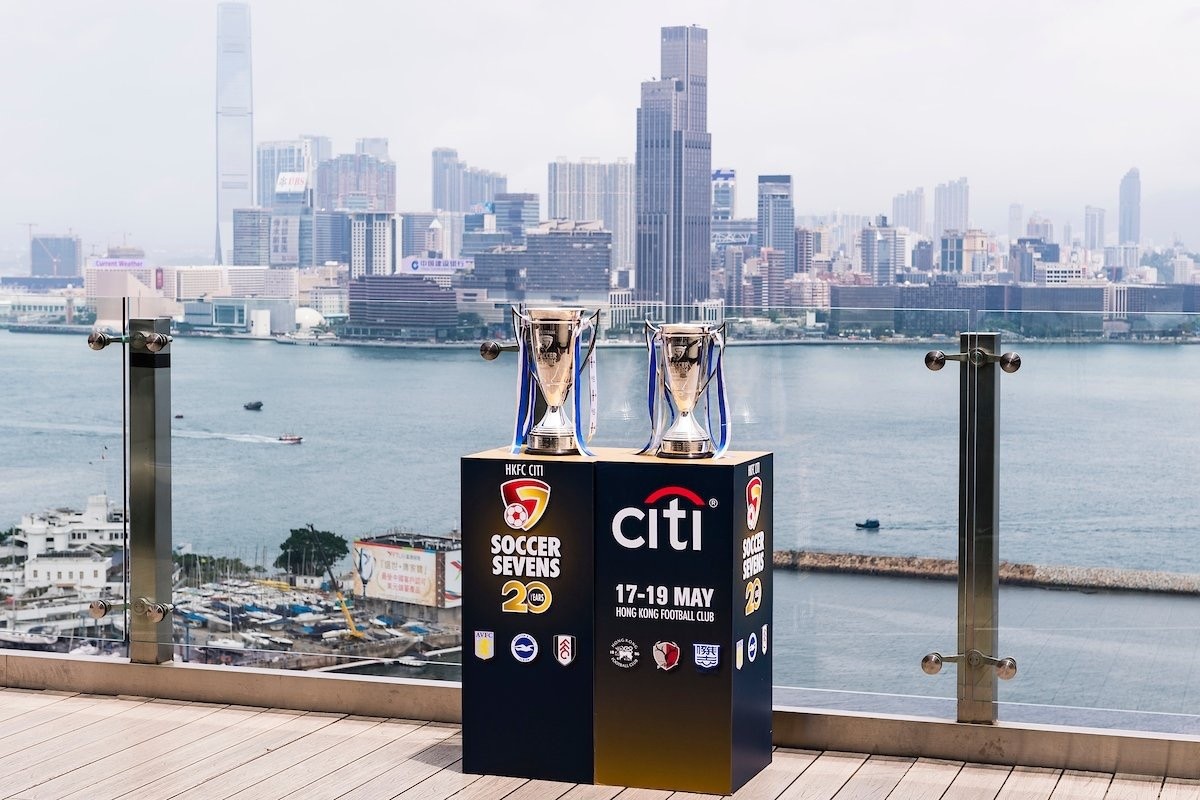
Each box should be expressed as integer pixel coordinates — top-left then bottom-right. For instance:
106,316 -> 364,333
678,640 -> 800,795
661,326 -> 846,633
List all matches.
511,304 -> 601,456
644,319 -> 727,459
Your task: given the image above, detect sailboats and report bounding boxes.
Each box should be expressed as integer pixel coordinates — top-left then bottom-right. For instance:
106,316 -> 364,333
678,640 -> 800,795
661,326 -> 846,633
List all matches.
69,542 -> 448,667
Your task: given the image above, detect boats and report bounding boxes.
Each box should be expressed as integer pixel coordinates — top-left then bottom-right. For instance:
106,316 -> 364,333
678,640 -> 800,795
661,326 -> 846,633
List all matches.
243,401 -> 263,411
856,519 -> 880,529
276,328 -> 341,346
279,433 -> 304,444
